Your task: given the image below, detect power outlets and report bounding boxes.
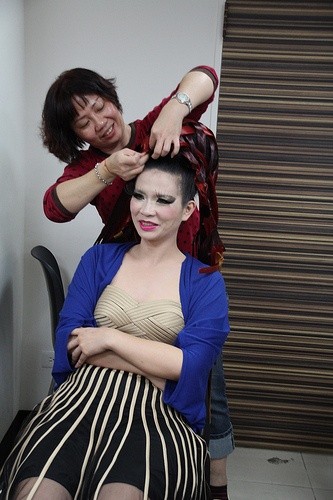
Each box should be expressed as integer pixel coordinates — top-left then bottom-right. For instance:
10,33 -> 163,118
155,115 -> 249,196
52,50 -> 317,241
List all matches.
40,351 -> 55,367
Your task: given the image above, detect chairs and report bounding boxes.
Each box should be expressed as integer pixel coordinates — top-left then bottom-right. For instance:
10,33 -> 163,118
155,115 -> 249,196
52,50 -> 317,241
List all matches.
30,245 -> 67,395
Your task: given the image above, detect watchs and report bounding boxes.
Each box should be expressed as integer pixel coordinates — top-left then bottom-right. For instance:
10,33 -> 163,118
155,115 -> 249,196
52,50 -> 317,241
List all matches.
170,92 -> 194,113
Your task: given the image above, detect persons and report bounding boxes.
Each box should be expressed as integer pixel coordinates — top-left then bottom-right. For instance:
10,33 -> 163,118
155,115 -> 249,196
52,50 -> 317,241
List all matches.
12,155 -> 229,499
42,66 -> 238,500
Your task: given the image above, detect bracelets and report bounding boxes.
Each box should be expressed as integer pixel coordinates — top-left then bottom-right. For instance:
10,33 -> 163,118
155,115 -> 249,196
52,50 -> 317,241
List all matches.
94,162 -> 111,186
